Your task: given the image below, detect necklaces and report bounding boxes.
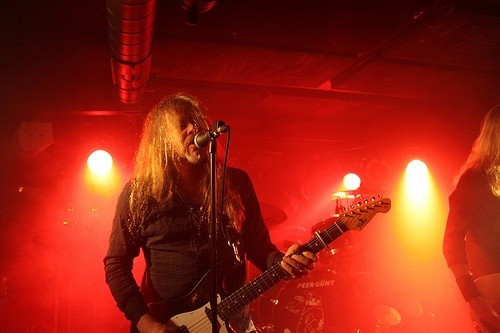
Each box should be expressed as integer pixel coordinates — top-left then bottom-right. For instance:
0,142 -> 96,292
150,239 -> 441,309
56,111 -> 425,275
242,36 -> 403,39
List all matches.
172,171 -> 217,238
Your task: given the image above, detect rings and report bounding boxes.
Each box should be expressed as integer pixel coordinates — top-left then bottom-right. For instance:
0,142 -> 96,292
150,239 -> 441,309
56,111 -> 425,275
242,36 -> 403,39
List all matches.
300,266 -> 306,273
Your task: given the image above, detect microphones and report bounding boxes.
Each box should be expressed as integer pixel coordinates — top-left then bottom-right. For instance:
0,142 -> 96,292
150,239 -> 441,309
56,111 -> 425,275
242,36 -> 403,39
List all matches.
194,119 -> 229,149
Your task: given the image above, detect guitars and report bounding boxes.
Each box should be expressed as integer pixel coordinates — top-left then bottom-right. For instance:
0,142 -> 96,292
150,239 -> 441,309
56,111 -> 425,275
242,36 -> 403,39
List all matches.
164,194 -> 390,333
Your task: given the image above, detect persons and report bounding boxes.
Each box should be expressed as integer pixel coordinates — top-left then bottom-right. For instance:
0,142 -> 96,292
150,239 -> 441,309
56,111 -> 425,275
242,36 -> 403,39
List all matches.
441,102 -> 499,332
101,89 -> 320,333
